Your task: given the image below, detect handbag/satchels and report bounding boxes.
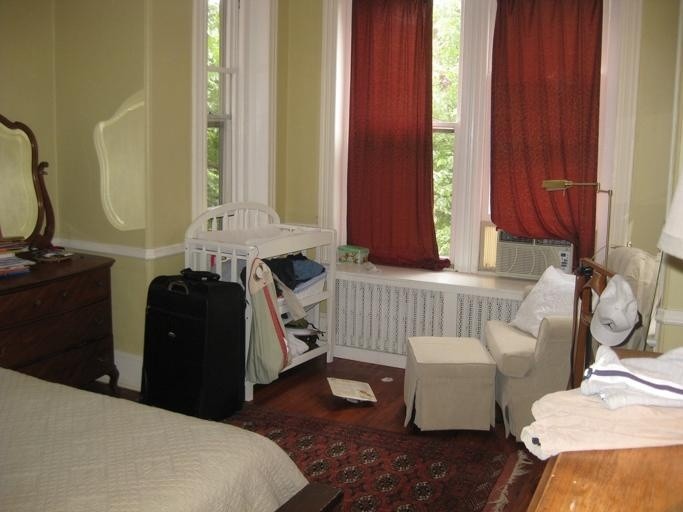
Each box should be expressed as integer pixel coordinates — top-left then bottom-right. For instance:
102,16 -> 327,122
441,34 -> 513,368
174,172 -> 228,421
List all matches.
245,257 -> 292,385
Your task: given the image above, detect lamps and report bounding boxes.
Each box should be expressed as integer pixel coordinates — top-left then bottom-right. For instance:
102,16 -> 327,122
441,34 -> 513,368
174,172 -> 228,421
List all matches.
542,178 -> 613,267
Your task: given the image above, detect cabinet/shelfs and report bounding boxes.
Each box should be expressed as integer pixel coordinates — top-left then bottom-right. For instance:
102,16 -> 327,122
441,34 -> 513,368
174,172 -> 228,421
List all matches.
186,203 -> 340,403
0,250 -> 122,391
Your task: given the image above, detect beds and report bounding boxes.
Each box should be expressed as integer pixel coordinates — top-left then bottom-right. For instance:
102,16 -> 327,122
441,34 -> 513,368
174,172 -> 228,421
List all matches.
1,363 -> 341,510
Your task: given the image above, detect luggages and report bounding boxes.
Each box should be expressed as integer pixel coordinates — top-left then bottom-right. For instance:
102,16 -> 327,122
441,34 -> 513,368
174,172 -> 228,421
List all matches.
140,267 -> 246,422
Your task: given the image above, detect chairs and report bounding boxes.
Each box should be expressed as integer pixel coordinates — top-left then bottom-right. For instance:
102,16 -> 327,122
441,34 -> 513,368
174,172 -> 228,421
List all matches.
482,242 -> 657,449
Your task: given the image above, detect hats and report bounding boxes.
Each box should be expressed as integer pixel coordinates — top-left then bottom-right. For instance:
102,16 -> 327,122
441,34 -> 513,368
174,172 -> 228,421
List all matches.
589,274 -> 638,347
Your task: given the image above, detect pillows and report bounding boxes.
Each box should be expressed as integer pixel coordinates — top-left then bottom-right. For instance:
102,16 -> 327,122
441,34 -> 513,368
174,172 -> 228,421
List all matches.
505,264 -> 584,338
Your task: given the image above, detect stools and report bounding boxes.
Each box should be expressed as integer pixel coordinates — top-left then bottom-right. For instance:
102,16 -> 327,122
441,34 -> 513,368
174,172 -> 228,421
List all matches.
403,336 -> 497,434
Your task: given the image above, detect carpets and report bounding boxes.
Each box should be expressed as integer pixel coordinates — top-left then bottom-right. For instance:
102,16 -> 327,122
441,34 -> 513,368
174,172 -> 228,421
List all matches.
203,405 -> 541,511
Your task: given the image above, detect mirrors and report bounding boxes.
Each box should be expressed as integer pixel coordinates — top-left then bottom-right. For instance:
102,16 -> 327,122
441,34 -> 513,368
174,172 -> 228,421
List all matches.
0,116 -> 57,257
